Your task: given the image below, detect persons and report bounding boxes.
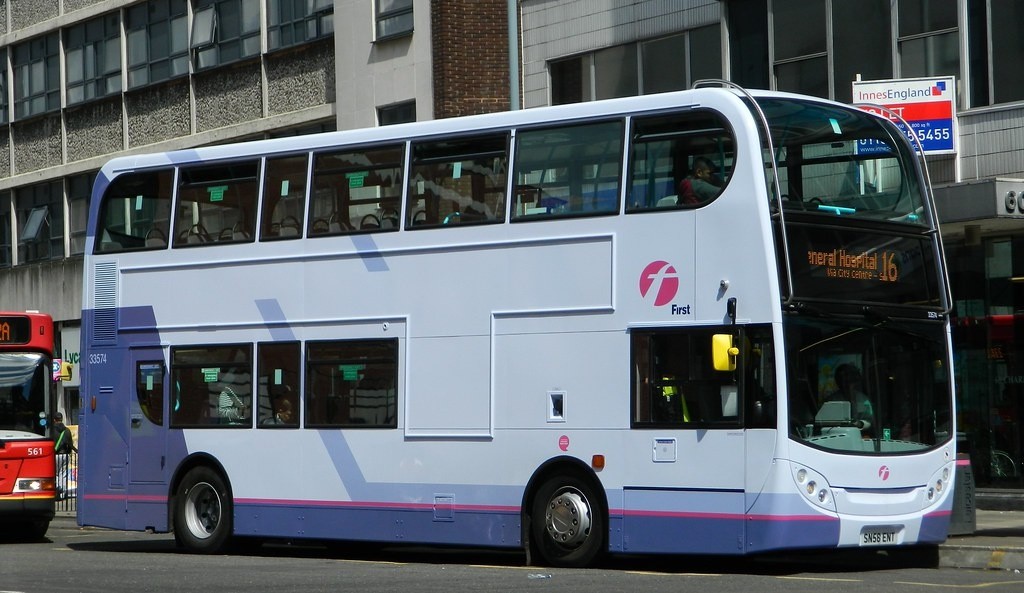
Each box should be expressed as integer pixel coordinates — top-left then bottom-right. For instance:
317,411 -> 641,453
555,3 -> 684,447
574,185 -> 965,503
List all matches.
218,372 -> 251,428
52,412 -> 73,502
263,395 -> 295,427
823,364 -> 874,436
677,157 -> 723,206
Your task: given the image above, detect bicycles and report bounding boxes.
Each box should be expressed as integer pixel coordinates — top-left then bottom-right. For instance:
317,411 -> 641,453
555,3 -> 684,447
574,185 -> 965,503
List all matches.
968,427 -> 1020,480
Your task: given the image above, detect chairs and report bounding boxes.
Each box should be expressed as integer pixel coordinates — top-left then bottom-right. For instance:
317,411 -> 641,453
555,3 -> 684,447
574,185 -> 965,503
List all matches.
99,210 -> 487,253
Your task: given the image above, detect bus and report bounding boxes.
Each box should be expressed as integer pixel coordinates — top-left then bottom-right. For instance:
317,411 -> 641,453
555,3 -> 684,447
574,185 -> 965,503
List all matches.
0,309 -> 75,542
77,77 -> 960,569
